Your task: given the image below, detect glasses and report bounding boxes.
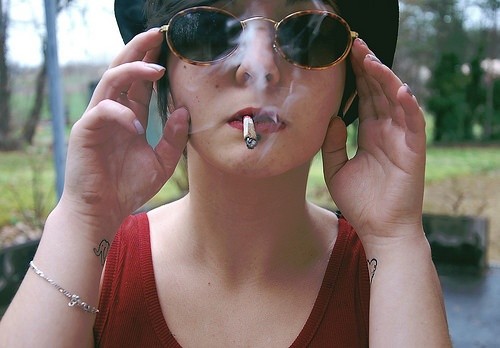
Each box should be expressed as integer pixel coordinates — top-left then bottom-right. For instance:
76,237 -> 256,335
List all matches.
158,6 -> 357,70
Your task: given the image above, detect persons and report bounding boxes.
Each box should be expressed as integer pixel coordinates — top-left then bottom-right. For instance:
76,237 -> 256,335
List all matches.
0,0 -> 455,348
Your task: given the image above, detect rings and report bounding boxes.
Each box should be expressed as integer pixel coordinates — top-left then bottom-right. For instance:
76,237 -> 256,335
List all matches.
120,91 -> 128,95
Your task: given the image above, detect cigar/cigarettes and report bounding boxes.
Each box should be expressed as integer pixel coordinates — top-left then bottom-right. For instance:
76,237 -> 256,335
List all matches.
243,116 -> 257,149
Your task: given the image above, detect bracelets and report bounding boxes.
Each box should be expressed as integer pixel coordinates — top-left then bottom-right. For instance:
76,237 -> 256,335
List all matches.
28,259 -> 100,316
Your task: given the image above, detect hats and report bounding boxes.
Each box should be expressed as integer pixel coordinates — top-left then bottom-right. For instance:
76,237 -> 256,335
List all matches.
113,0 -> 400,127
